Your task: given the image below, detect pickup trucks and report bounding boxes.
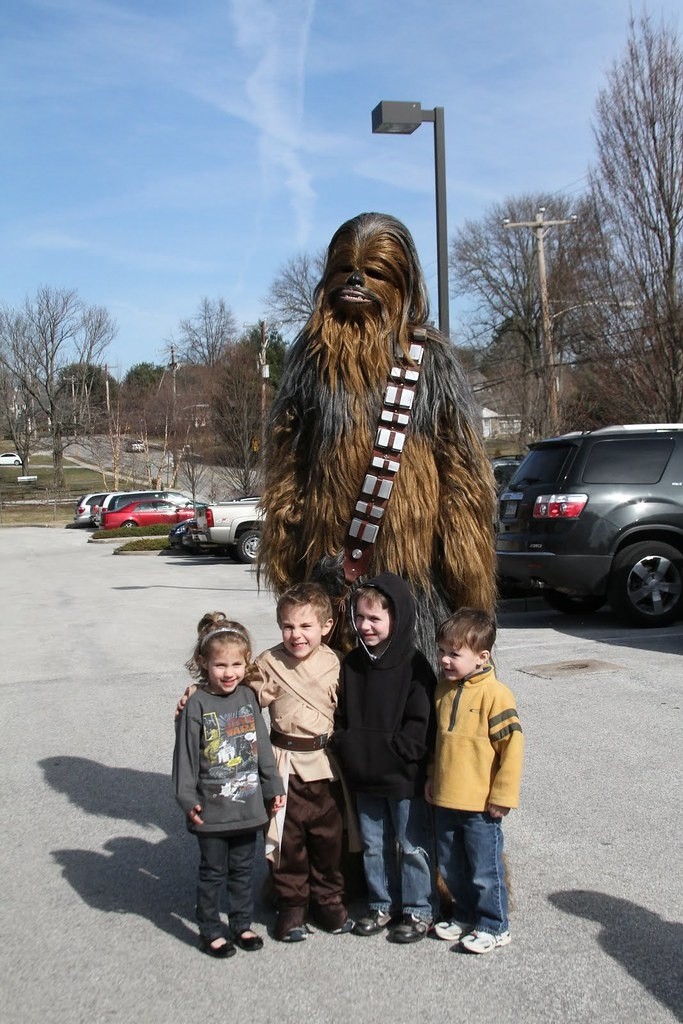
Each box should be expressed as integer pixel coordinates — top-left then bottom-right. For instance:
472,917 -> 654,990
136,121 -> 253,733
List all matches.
195,499 -> 269,565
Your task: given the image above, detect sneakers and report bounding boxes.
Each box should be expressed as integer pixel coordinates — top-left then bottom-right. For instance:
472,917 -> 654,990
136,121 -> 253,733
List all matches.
435,916 -> 475,940
328,918 -> 355,933
354,910 -> 401,936
460,929 -> 511,953
281,923 -> 308,942
394,913 -> 434,942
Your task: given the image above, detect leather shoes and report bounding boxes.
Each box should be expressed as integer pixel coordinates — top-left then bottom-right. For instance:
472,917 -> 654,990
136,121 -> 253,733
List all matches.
199,936 -> 237,958
229,925 -> 263,950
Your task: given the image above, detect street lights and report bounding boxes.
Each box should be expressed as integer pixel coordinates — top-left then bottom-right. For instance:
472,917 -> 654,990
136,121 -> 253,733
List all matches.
538,299 -> 638,439
171,403 -> 210,489
372,101 -> 449,342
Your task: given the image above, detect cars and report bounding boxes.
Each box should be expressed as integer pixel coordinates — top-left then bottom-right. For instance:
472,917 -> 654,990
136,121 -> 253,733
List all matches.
492,455 -> 527,487
74,491 -> 123,529
126,439 -> 146,453
168,518 -> 216,556
0,452 -> 31,466
98,498 -> 198,531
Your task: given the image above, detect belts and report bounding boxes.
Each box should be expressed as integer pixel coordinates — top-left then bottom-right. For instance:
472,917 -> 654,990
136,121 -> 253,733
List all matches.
270,727 -> 328,751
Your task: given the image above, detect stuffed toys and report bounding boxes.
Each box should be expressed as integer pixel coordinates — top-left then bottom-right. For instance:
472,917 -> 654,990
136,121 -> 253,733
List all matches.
263,211 -> 496,669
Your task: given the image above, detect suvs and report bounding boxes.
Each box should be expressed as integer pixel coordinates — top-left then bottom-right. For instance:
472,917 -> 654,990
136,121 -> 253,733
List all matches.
492,423 -> 683,628
92,489 -> 209,528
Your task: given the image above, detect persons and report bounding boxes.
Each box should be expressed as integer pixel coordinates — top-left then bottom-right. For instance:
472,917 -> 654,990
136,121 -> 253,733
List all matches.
326,571 -> 443,943
174,580 -> 355,940
421,604 -> 526,954
173,610 -> 286,958
